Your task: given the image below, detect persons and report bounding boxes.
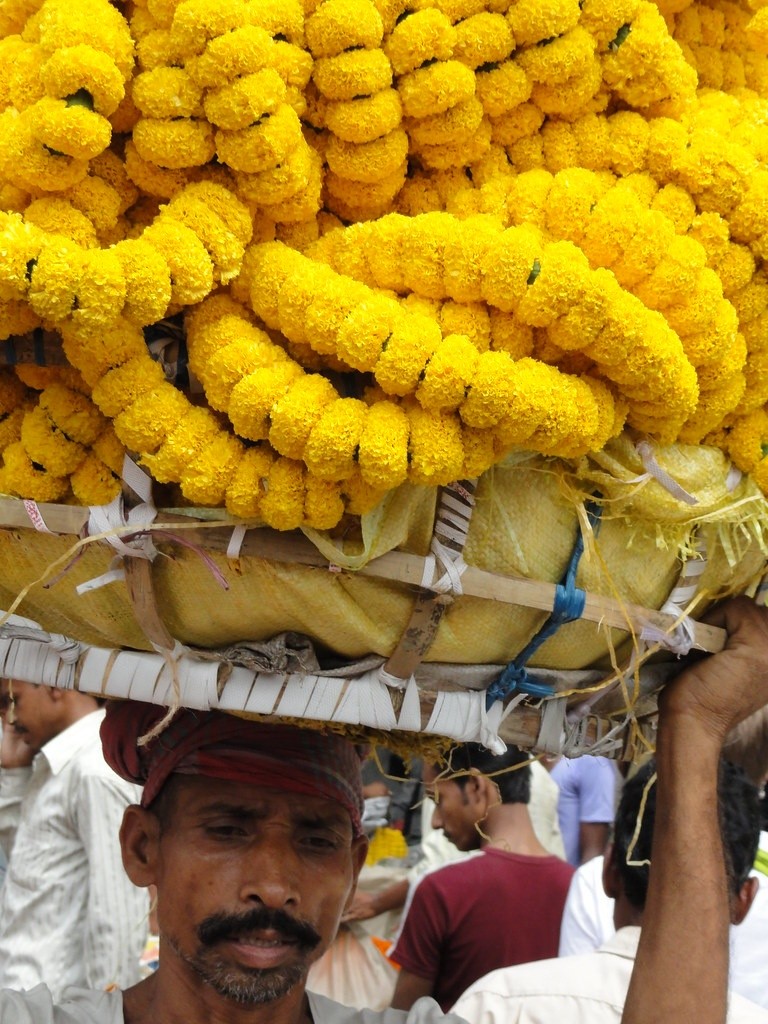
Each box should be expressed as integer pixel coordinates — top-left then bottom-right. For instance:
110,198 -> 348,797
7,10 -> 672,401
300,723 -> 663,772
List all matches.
0,594 -> 768,1023
342,737 -> 763,1014
0,678 -> 149,991
